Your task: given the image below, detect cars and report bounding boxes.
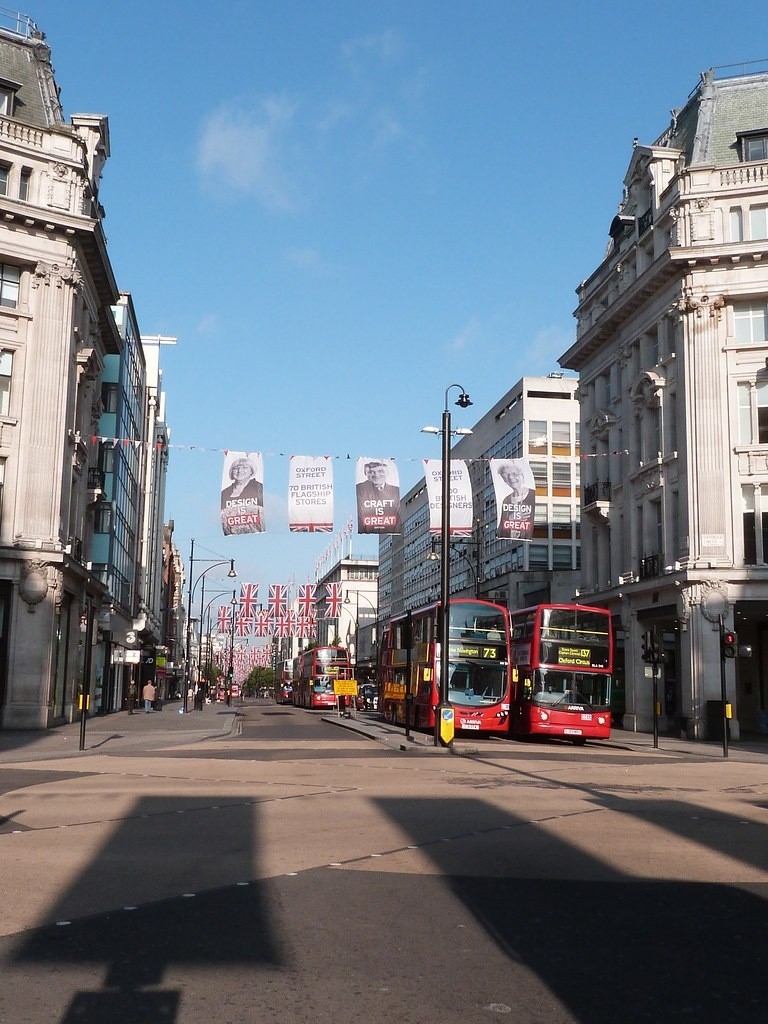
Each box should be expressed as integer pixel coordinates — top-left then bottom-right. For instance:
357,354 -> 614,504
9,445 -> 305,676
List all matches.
357,684 -> 377,712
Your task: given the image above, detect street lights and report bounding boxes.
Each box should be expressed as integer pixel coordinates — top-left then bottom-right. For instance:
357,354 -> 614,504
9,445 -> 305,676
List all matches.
426,517 -> 481,600
420,383 -> 475,747
345,576 -> 381,698
196,575 -> 238,712
182,537 -> 238,714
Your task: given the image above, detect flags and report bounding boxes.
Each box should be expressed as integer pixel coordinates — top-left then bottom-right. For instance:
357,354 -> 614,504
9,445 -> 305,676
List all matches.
315,520 -> 353,575
218,645 -> 271,684
217,582 -> 342,638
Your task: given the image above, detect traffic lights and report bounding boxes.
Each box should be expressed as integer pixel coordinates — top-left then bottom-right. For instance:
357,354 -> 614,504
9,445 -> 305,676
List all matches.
641,632 -> 650,662
229,667 -> 234,678
650,635 -> 662,664
724,632 -> 738,657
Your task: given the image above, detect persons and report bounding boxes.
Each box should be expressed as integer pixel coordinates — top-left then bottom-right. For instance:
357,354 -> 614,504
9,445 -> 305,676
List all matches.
356,462 -> 402,534
487,625 -> 501,641
221,457 -> 263,534
196,688 -> 203,711
188,688 -> 193,702
498,463 -> 535,541
154,685 -> 161,710
125,680 -> 138,715
143,680 -> 155,714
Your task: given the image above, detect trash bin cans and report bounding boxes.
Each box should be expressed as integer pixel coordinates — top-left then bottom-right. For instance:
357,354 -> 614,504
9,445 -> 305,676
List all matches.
339,697 -> 345,711
194,694 -> 204,710
706,699 -> 732,742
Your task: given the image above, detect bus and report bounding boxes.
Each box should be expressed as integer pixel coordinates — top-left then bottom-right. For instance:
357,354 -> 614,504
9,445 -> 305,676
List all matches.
378,598 -> 520,737
293,645 -> 352,709
274,658 -> 294,705
477,603 -> 613,746
